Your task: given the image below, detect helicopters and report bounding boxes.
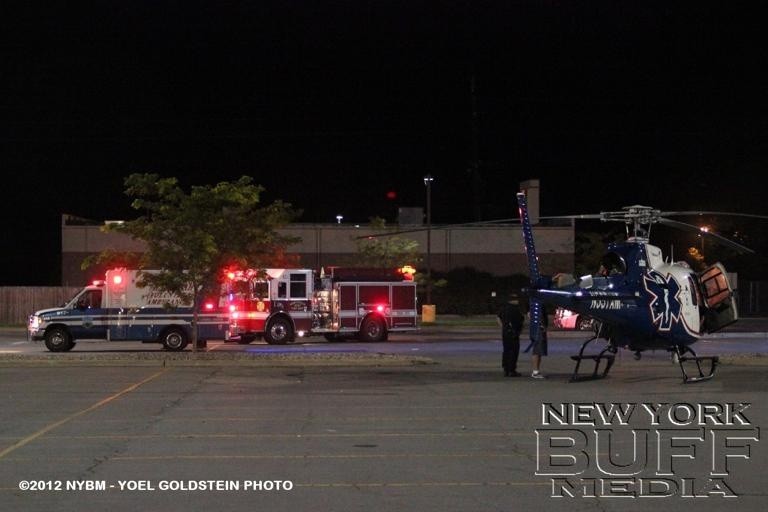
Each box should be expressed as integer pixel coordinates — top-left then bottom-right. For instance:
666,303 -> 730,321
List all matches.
349,192 -> 768,385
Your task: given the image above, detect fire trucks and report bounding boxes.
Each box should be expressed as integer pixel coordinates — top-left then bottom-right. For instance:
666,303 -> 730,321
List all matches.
230,265 -> 419,344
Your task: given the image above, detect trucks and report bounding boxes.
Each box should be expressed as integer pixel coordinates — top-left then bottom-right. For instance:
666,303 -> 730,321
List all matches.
28,266 -> 230,351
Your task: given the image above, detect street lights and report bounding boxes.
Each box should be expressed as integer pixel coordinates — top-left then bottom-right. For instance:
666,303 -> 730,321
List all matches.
422,176 -> 439,303
336,215 -> 343,223
698,226 -> 708,257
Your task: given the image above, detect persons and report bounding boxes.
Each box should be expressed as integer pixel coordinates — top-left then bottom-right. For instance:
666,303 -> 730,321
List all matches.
528,298 -> 549,380
496,290 -> 525,378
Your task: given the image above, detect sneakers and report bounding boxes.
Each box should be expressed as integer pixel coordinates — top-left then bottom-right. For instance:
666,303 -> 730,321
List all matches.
531,371 -> 545,380
503,370 -> 523,377
